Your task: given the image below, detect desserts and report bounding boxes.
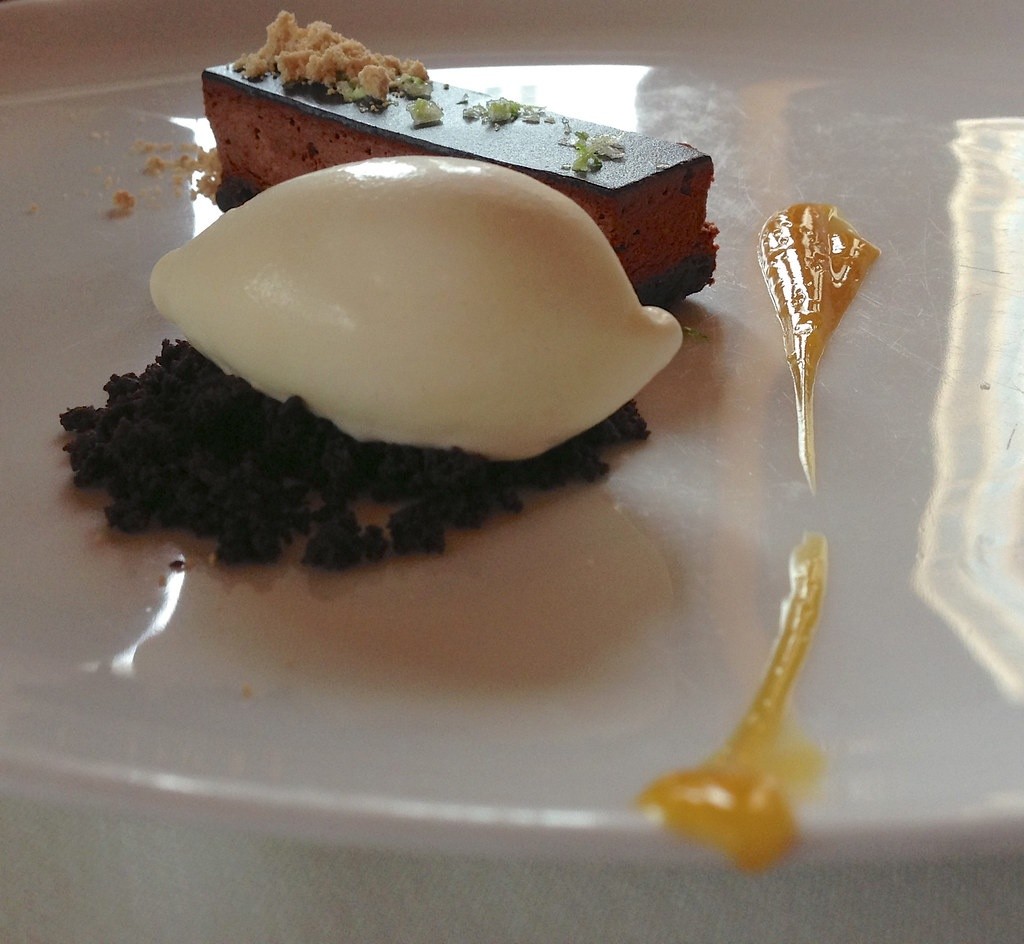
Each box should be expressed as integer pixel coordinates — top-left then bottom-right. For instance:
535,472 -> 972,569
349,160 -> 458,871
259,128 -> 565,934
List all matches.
57,155 -> 683,570
201,10 -> 722,313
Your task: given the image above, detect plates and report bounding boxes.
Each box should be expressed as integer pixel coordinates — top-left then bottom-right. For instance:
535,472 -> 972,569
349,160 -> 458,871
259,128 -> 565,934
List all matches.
0,0 -> 1024,869
